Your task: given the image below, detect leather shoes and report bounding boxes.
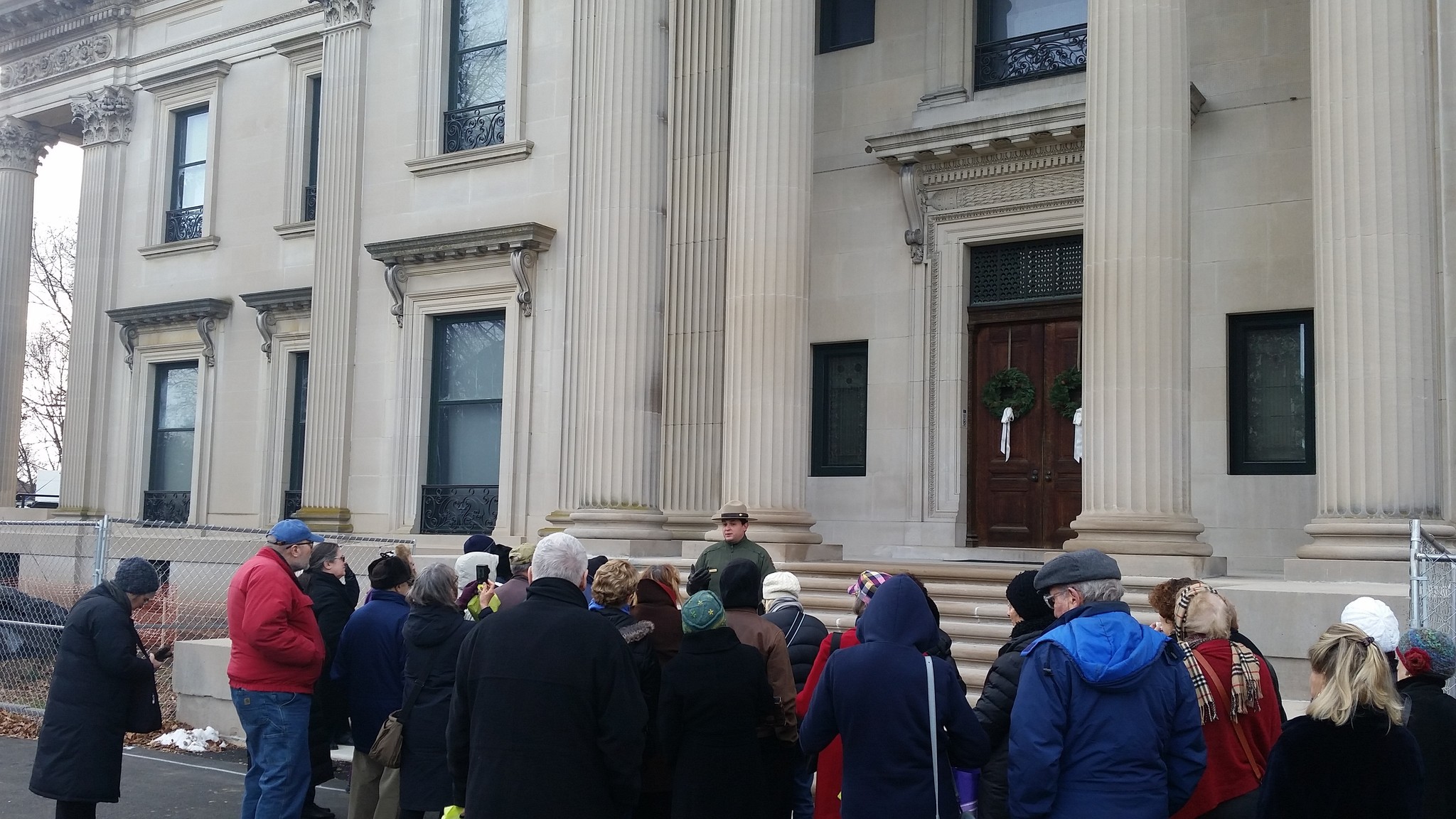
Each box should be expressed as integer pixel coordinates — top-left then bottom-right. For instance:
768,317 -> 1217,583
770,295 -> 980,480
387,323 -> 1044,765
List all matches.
313,800 -> 331,814
303,804 -> 336,819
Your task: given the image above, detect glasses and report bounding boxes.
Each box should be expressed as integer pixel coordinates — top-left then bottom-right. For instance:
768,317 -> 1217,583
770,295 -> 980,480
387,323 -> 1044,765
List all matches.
333,555 -> 345,562
285,541 -> 314,549
1043,586 -> 1073,610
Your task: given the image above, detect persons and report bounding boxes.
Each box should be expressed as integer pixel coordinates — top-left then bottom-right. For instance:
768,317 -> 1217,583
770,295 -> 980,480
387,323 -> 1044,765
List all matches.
399,562 -> 496,819
1144,577 -> 1456,818
27,557 -> 165,817
892,566 -> 1066,818
227,520 -> 326,819
330,557 -> 418,819
361,530 -> 697,681
797,571 -> 992,817
715,557 -> 892,818
443,530 -> 650,818
583,558 -> 667,818
686,502 -> 778,601
1005,547 -> 1207,819
293,541 -> 360,819
657,589 -> 785,818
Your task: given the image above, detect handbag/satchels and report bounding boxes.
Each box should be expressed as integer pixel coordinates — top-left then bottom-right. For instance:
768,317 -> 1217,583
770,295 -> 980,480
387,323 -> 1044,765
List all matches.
120,656 -> 163,733
369,709 -> 407,769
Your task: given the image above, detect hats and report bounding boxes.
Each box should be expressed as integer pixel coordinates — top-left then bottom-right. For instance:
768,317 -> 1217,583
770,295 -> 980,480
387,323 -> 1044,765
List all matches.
1005,569 -> 1055,621
266,519 -> 325,546
1035,550 -> 1122,589
587,556 -> 610,585
463,535 -> 496,556
368,556 -> 411,590
1396,629 -> 1456,679
721,558 -> 762,609
763,572 -> 802,600
509,542 -> 537,565
846,569 -> 895,605
681,590 -> 727,631
711,500 -> 759,523
1340,596 -> 1400,654
115,558 -> 160,595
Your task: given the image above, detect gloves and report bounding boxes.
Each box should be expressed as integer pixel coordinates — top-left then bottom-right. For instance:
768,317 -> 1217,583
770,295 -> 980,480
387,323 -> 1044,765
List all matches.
686,564 -> 712,593
458,578 -> 480,608
